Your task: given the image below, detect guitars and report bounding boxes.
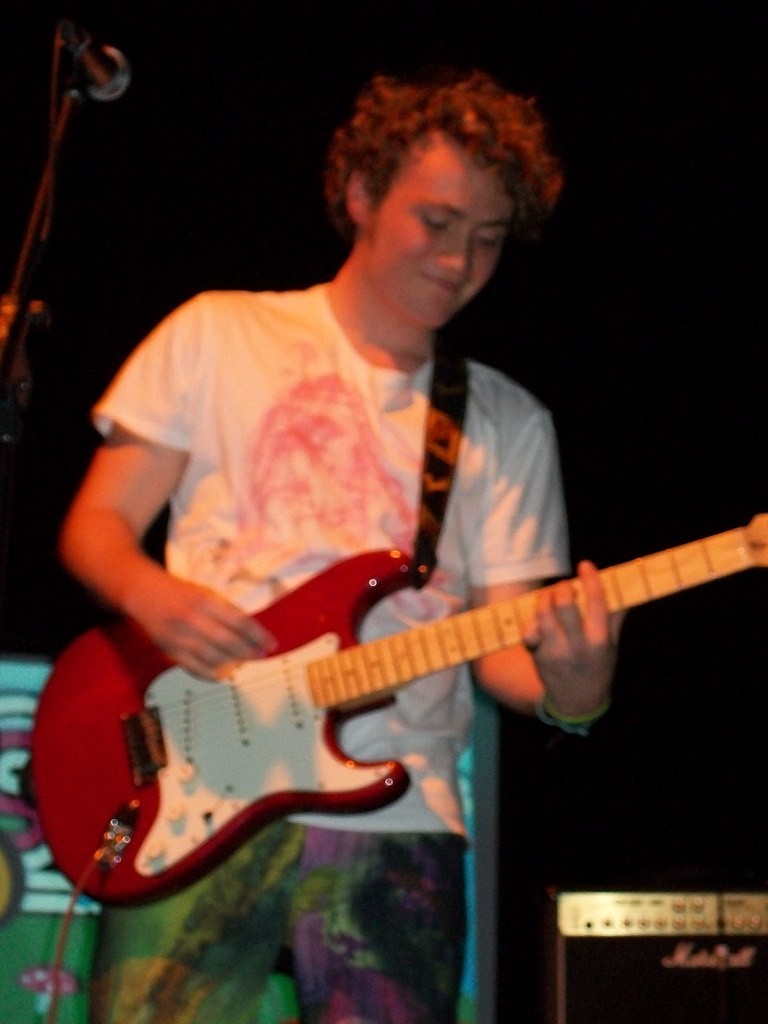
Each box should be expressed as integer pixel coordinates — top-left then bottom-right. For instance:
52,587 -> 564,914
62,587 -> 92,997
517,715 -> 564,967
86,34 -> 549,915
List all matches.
33,509 -> 768,908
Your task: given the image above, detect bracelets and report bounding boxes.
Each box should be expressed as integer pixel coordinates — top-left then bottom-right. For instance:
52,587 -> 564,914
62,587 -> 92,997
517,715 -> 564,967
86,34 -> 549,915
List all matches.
536,696 -> 605,737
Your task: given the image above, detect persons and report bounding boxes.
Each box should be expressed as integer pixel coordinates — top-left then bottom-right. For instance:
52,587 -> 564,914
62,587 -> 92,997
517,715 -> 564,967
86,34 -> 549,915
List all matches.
61,72 -> 622,1024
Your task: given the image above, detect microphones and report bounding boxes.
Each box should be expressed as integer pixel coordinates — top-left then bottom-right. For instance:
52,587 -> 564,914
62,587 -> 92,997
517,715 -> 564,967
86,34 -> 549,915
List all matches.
38,8 -> 134,103
